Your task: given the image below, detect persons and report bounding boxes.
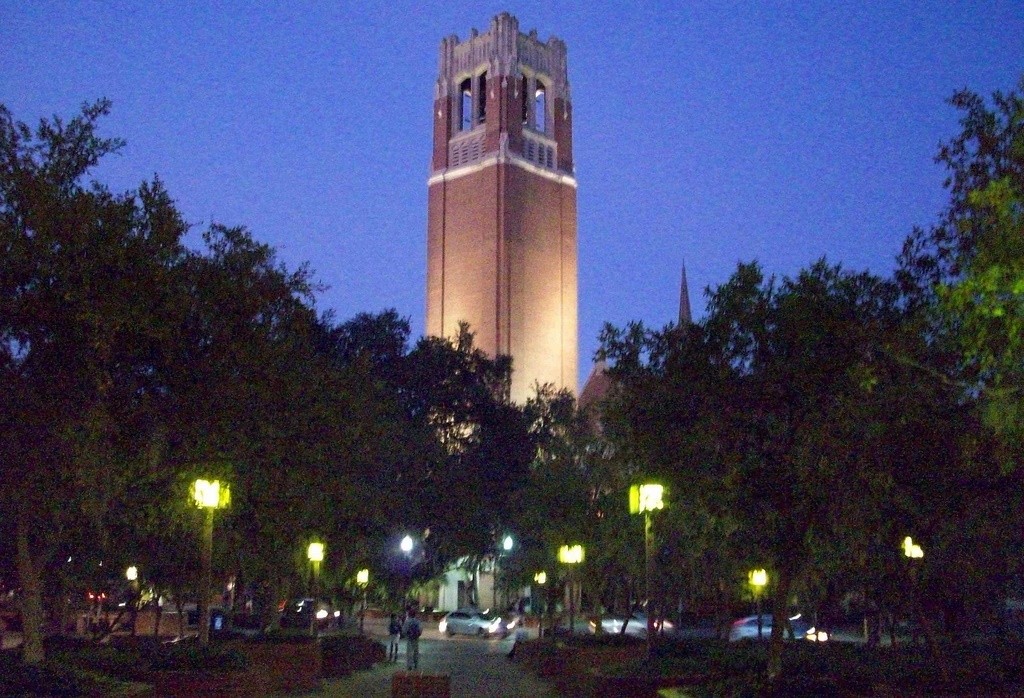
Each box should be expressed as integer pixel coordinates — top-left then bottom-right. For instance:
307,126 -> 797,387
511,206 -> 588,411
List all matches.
388,613 -> 402,659
504,619 -> 529,659
401,609 -> 423,670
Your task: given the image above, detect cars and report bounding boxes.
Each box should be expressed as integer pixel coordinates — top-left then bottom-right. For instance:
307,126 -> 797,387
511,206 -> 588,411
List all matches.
438,609 -> 513,640
729,614 -> 813,642
587,613 -> 677,635
192,594 -> 346,632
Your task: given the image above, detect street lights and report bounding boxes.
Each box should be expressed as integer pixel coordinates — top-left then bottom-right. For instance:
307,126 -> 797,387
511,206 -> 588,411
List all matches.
534,570 -> 545,639
308,541 -> 325,639
749,568 -> 768,639
640,482 -> 665,661
193,478 -> 222,643
401,536 -> 414,617
504,535 -> 513,607
558,544 -> 584,633
357,569 -> 367,632
